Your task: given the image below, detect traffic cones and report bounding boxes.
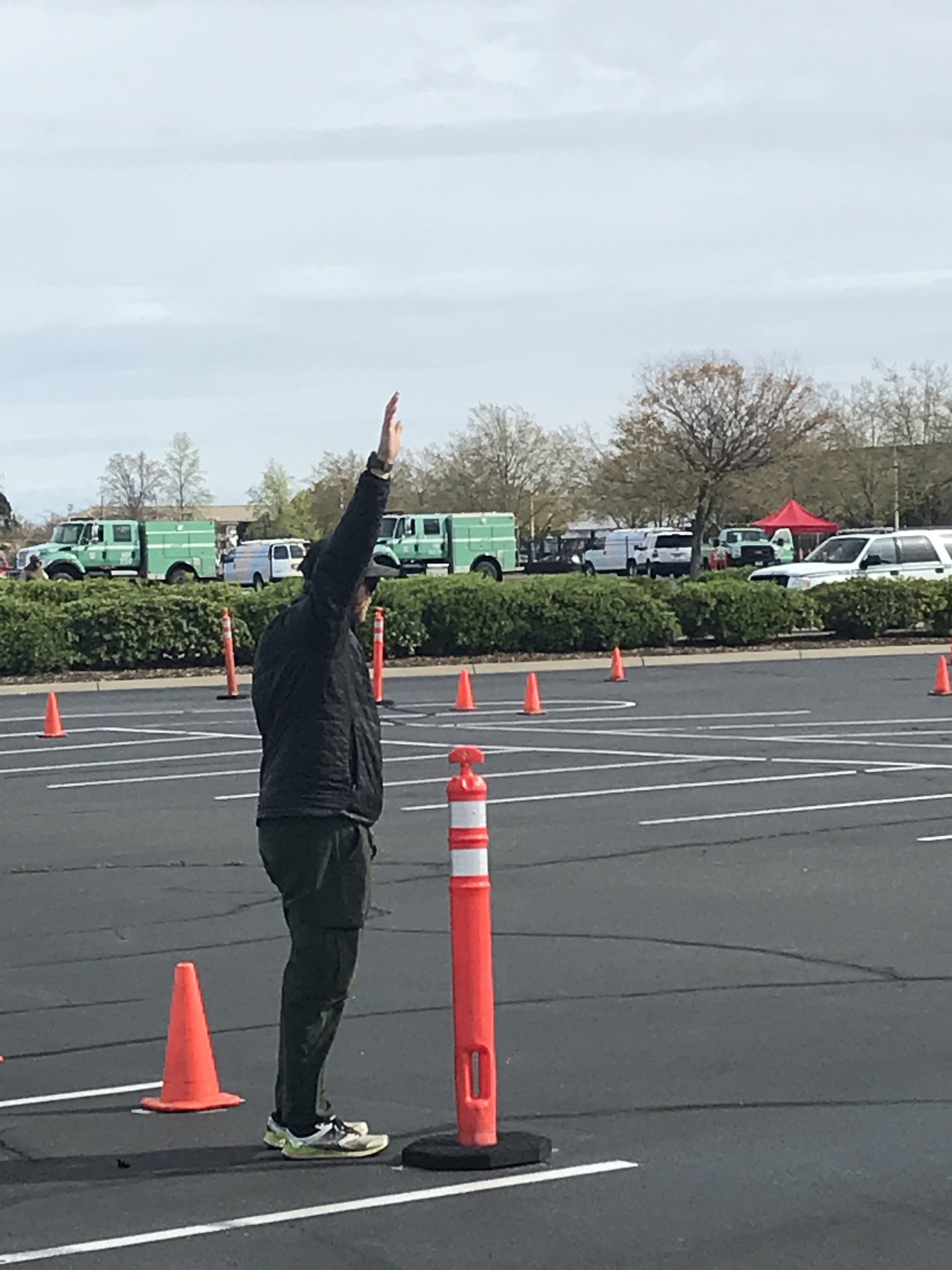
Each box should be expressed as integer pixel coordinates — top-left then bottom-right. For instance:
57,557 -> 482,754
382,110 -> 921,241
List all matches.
139,962 -> 242,1113
448,670 -> 479,711
514,672 -> 548,716
602,645 -> 629,681
926,654 -> 952,696
37,691 -> 68,738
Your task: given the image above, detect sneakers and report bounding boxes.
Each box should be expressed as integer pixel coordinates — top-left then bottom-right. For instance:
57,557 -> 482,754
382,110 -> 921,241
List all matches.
264,1111 -> 368,1151
282,1115 -> 390,1158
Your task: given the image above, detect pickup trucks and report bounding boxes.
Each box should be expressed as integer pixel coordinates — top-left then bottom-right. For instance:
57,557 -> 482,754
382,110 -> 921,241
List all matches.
700,528 -> 775,570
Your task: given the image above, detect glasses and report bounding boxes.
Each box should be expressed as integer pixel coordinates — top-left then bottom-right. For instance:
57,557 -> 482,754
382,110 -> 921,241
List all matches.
363,578 -> 382,590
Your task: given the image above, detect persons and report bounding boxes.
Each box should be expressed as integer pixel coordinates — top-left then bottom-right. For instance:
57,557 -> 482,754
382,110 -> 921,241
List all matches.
710,548 -> 732,571
18,555 -> 49,581
799,543 -> 844,562
251,390 -> 402,1159
0,541 -> 11,579
774,538 -> 785,566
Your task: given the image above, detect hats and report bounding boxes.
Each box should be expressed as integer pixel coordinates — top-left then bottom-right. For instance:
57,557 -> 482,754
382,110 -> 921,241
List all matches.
296,540 -> 401,577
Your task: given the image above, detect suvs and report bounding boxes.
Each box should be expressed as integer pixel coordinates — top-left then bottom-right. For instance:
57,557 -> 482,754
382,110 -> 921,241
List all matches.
625,531 -> 694,579
746,527 -> 952,596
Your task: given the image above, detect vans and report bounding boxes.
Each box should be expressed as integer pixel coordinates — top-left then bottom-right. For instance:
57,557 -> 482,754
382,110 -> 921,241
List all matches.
229,538 -> 309,592
582,527 -> 680,577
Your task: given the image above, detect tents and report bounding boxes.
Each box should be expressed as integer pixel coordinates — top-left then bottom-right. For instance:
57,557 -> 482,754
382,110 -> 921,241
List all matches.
752,499 -> 838,545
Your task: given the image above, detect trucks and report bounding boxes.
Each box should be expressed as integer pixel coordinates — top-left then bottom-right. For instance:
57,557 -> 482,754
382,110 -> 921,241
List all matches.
374,510 -> 525,586
15,517 -> 220,585
